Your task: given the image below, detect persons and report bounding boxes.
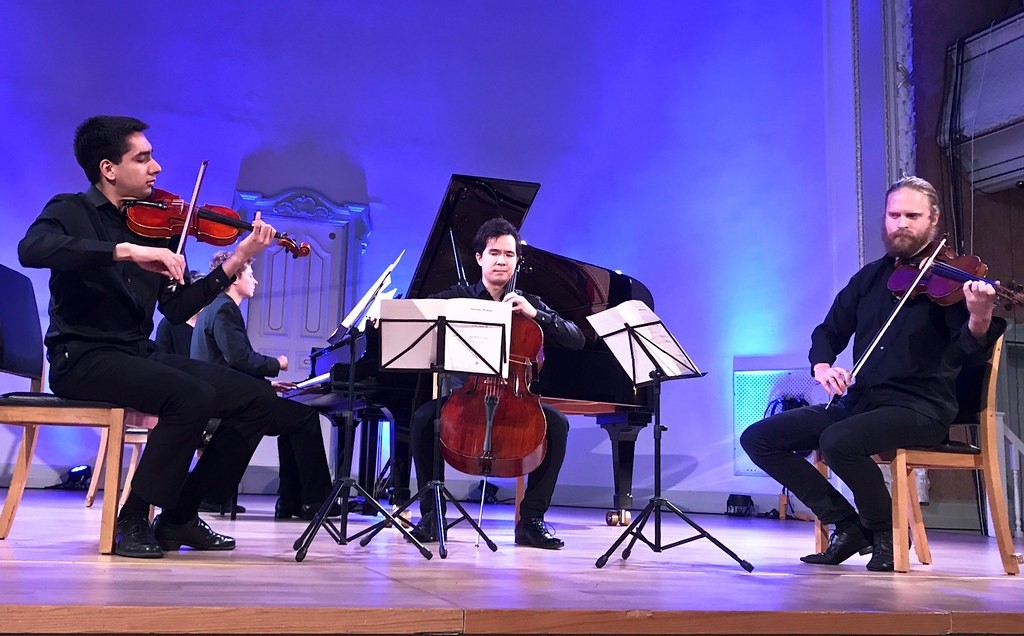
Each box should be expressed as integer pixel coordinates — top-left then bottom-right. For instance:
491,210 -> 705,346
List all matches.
741,176 -> 1001,571
17,114 -> 278,558
190,248 -> 358,523
156,269 -> 246,514
404,218 -> 571,550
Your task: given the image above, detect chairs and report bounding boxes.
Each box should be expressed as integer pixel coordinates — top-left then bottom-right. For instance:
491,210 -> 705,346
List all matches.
817,332 -> 1021,575
0,265 -> 153,555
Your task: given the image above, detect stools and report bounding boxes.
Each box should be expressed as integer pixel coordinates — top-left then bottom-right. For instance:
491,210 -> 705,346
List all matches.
205,418 -> 238,521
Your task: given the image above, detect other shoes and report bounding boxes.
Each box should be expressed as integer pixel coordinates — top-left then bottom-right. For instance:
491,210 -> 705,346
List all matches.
199,498 -> 246,513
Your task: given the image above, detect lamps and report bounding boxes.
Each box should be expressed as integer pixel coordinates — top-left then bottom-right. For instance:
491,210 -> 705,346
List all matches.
478,480 -> 499,505
727,493 -> 753,517
68,466 -> 92,489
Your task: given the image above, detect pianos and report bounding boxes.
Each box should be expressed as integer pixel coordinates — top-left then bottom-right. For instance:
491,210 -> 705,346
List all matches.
283,173 -> 655,526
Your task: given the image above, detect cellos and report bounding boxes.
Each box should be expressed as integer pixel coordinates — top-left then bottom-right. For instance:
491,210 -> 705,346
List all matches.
439,240 -> 547,548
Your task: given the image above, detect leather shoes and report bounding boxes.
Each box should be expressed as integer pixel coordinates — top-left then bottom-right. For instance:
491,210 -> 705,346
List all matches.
150,513 -> 236,552
403,511 -> 448,542
274,496 -> 359,520
800,520 -> 874,566
515,517 -> 565,549
114,514 -> 163,558
866,527 -> 912,571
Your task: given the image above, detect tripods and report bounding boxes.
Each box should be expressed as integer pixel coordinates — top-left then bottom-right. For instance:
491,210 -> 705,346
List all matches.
293,271 -> 434,561
595,320 -> 755,573
360,318 -> 509,556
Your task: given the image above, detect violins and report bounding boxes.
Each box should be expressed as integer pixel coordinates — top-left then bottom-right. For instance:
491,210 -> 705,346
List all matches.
887,240 -> 1024,312
125,187 -> 311,260
